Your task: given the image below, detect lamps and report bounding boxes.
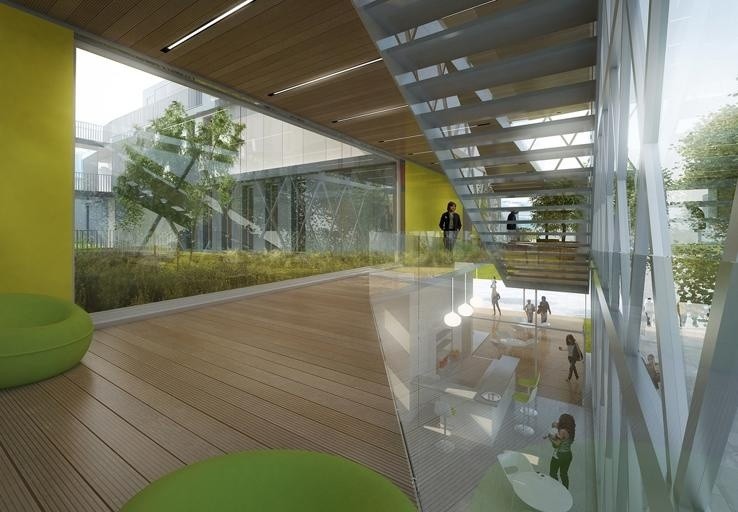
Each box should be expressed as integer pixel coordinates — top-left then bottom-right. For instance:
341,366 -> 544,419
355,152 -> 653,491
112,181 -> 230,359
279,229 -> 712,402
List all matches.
496,452 -> 534,483
0,291 -> 94,389
409,316 -> 551,452
118,449 -> 418,511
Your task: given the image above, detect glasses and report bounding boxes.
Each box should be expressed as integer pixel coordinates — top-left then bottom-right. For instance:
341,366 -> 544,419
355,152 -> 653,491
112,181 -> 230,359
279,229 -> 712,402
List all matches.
444,277 -> 462,327
457,271 -> 474,317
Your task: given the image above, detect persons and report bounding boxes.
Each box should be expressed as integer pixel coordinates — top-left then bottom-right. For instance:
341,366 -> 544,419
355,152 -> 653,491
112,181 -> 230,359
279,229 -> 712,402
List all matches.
439,201 -> 462,264
645,354 -> 661,390
492,290 -> 502,316
524,300 -> 535,322
506,211 -> 520,232
559,334 -> 583,383
644,297 -> 654,327
537,296 -> 551,324
547,414 -> 576,490
677,301 -> 688,329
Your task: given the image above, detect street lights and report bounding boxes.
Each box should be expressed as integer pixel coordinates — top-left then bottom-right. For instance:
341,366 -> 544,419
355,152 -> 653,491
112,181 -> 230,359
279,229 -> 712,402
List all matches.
571,344 -> 583,361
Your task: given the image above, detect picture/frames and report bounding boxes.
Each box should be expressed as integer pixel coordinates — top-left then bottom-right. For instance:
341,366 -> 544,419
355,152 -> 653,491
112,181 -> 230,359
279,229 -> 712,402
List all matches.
509,472 -> 574,512
